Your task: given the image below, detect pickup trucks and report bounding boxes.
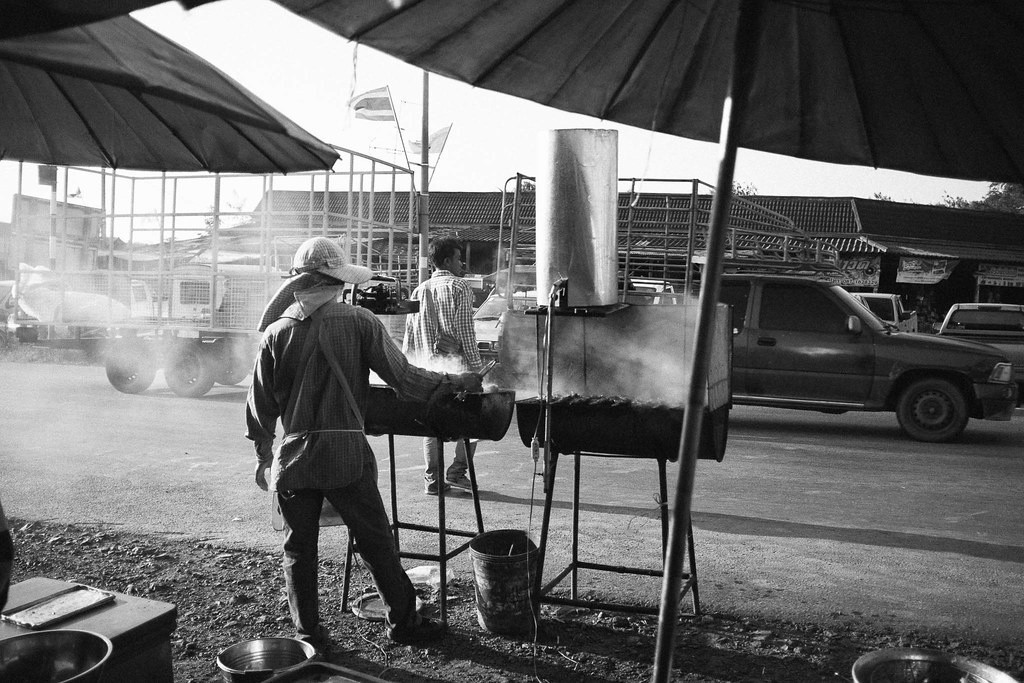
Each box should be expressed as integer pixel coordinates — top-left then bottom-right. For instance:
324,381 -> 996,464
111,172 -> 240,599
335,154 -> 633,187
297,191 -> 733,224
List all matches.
848,293 -> 919,335
930,303 -> 1024,410
715,272 -> 1022,444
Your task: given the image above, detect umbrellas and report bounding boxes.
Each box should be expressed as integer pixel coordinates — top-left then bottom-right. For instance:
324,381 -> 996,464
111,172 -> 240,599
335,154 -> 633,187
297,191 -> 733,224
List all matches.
271,0 -> 1024,683
0,13 -> 338,172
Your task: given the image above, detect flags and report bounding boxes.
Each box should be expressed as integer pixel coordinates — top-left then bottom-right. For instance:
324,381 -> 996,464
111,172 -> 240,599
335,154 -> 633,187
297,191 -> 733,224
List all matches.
347,87 -> 397,122
407,127 -> 449,168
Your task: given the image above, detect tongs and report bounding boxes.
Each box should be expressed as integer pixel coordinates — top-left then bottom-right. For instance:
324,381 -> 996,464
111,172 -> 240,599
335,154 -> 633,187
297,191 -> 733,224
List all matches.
453,360 -> 496,401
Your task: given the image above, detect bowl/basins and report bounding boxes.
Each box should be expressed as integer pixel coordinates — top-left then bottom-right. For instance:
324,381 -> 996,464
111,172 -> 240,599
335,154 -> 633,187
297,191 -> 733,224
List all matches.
0,629 -> 113,683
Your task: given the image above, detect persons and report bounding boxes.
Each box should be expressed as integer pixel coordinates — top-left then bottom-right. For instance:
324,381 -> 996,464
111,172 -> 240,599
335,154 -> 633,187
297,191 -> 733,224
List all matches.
244,237 -> 483,643
403,238 -> 484,494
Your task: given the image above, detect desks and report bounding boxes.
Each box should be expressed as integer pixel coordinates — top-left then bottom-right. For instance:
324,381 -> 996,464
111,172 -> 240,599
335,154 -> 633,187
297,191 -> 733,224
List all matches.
0,575 -> 178,682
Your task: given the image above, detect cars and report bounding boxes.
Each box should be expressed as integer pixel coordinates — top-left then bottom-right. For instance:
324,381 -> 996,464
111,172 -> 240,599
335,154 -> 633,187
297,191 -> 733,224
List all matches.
454,272 -> 678,368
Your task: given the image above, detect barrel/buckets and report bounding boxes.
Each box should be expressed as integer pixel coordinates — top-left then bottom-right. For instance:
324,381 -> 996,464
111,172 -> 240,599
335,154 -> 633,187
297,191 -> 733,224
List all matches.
851,648 -> 1018,683
216,634 -> 316,683
468,529 -> 540,634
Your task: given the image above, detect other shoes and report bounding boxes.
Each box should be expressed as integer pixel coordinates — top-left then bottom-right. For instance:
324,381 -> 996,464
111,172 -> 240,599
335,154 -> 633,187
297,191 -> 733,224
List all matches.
386,617 -> 446,646
447,476 -> 479,490
426,481 -> 450,495
307,631 -> 332,651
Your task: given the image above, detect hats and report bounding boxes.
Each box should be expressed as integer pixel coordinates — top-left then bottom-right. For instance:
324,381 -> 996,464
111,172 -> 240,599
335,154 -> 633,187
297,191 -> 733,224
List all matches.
257,237 -> 374,332
